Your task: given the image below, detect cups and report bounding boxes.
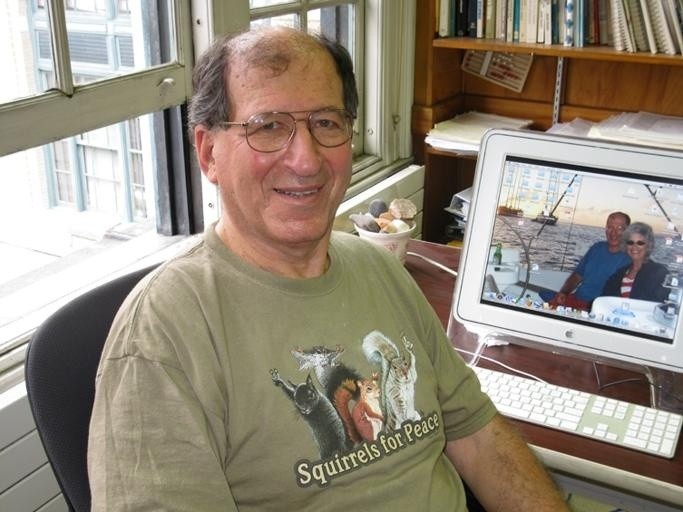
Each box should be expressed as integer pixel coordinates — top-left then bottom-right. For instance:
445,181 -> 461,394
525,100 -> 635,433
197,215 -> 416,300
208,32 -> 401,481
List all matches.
352,218 -> 417,269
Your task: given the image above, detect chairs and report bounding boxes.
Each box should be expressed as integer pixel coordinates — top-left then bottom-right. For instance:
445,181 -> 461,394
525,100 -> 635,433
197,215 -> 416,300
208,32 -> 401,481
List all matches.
24,262 -> 484,512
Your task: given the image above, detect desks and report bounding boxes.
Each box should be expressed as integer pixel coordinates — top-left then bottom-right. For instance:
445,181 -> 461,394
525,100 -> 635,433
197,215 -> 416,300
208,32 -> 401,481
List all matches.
344,231 -> 683,512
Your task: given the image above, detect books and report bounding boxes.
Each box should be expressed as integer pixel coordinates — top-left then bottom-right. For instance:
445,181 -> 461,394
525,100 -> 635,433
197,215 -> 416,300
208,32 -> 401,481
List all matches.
435,0 -> 683,56
423,110 -> 683,156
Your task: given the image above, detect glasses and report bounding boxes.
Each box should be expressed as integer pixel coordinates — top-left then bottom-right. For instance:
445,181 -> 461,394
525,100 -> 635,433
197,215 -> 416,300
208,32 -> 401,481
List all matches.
192,109 -> 353,153
607,225 -> 625,230
625,240 -> 645,245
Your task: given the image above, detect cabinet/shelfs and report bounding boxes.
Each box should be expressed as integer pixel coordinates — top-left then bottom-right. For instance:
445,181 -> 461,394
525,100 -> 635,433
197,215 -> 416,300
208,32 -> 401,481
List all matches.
413,0 -> 683,236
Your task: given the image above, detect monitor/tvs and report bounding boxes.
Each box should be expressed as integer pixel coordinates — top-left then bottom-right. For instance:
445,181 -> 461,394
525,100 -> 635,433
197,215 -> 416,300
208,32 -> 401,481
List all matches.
446,127 -> 683,416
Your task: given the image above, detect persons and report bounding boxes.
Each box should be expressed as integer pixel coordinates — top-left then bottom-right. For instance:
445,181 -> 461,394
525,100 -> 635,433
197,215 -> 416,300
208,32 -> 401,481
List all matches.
549,212 -> 671,312
87,27 -> 572,512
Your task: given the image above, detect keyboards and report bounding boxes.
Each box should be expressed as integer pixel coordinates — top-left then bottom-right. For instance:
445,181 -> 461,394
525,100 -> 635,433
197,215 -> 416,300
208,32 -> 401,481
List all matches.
466,363 -> 683,458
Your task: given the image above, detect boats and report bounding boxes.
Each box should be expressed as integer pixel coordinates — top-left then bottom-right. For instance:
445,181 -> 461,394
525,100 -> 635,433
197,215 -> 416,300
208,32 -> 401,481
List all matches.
498,206 -> 522,217
533,211 -> 558,225
